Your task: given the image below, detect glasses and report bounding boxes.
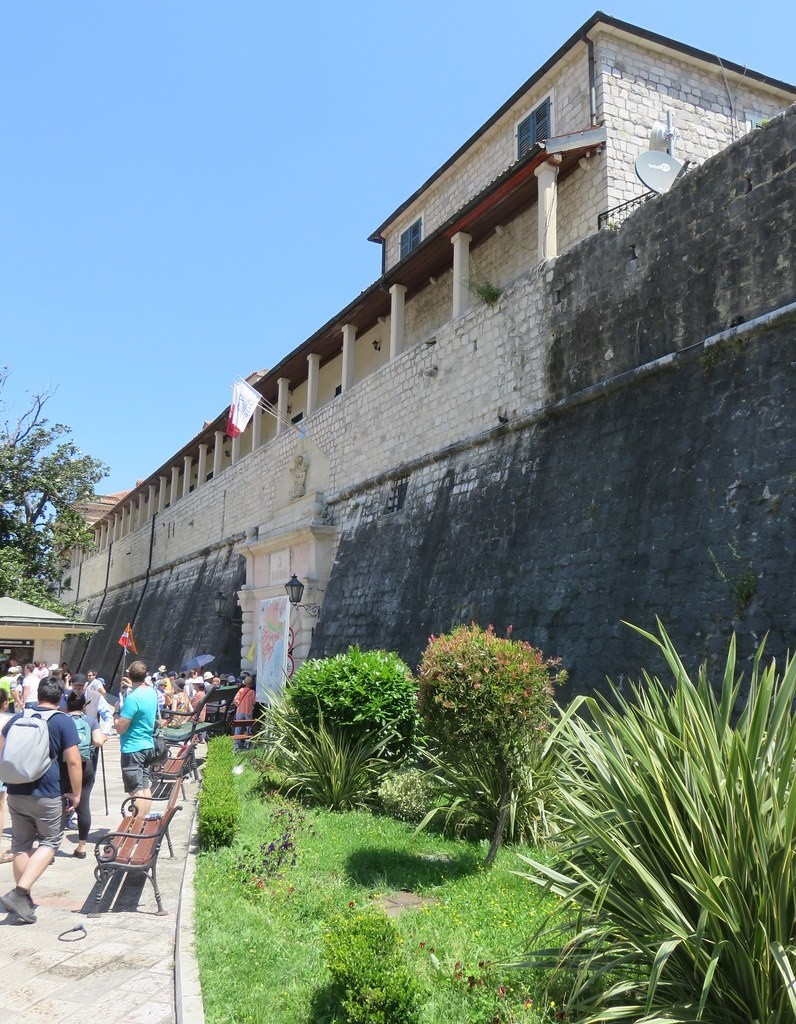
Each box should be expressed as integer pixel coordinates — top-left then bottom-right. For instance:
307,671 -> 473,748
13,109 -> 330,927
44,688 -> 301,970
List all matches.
87,674 -> 92,677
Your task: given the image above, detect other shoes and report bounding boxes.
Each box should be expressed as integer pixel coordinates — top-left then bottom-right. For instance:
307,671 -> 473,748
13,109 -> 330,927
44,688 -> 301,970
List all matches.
0,851 -> 14,863
74,849 -> 86,858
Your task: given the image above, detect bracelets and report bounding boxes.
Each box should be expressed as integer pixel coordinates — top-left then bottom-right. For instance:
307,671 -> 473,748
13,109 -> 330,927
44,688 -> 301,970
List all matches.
113,712 -> 119,716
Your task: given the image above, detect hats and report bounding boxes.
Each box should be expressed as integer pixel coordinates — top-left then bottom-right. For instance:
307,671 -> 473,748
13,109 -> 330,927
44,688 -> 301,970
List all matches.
98,678 -> 106,685
228,676 -> 236,683
192,676 -> 204,683
48,664 -> 59,671
158,664 -> 168,672
72,674 -> 87,686
168,671 -> 177,676
8,667 -> 20,673
203,671 -> 214,680
172,678 -> 186,691
242,676 -> 254,686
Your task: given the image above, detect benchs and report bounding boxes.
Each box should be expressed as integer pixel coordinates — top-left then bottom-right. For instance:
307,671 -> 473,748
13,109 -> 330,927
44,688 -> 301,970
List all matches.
88,685 -> 242,917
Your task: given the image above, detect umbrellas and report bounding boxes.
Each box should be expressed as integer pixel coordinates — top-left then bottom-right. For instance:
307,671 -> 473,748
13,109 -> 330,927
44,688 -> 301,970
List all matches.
179,653 -> 216,671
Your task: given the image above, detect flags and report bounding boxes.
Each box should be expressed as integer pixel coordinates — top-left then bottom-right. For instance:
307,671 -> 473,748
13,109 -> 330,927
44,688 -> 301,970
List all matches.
118,623 -> 138,655
226,378 -> 262,438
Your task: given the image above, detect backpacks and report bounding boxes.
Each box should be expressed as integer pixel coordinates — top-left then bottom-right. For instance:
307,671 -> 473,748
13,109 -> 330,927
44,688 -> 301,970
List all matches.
62,714 -> 92,762
0,708 -> 66,784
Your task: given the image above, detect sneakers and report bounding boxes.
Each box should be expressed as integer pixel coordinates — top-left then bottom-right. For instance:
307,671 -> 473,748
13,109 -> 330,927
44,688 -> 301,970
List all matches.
0,889 -> 37,923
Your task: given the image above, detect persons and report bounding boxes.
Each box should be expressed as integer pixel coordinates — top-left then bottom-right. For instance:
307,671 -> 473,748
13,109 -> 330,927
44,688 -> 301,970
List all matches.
113,661 -> 158,817
0,676 -> 83,923
0,653 -> 256,864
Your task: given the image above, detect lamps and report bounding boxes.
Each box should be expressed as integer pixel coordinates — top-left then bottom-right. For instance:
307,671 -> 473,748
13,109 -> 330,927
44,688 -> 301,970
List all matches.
285,574 -> 304,605
225,450 -> 229,457
214,591 -> 227,616
372,340 -> 379,350
